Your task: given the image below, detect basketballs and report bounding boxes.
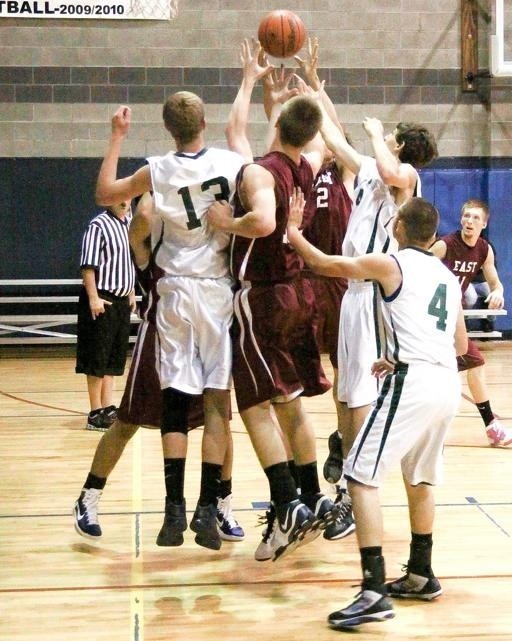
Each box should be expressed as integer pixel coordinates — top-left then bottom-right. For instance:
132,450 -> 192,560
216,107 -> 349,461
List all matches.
258,9 -> 305,58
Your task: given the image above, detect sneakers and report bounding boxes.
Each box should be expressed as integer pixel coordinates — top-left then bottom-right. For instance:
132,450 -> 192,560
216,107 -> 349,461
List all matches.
73,488 -> 103,541
385,560 -> 442,601
86,405 -> 119,431
156,492 -> 245,550
323,431 -> 343,484
255,485 -> 355,562
328,577 -> 395,627
485,418 -> 512,447
